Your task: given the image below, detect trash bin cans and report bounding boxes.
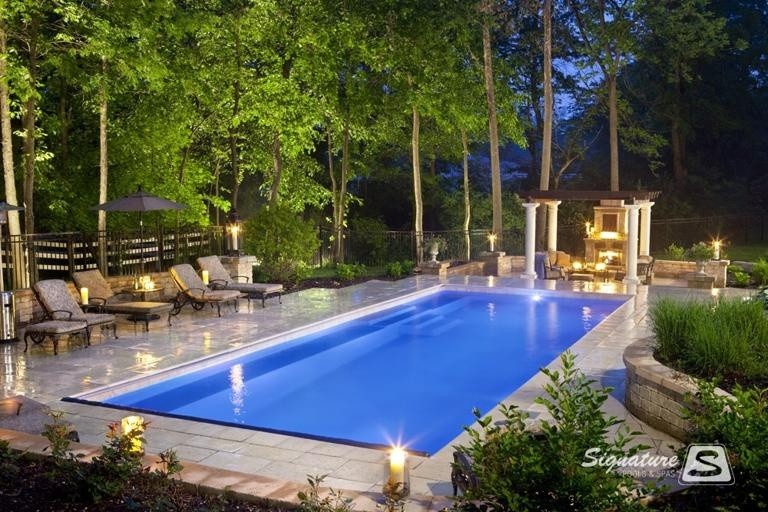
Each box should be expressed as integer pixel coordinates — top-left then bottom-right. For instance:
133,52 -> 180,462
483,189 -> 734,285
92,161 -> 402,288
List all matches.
0,291 -> 21,344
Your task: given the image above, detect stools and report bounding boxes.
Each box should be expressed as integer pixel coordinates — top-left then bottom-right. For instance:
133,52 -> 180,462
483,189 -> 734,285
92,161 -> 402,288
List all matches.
22,320 -> 89,357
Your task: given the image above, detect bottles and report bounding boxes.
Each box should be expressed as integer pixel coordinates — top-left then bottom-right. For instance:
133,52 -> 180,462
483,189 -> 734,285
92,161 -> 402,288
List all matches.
81,287 -> 88,305
133,275 -> 155,290
201,271 -> 209,285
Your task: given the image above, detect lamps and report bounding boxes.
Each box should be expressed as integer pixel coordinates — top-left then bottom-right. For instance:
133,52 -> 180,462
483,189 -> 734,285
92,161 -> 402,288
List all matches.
711,239 -> 720,261
486,231 -> 496,252
223,207 -> 242,257
118,414 -> 144,464
383,446 -> 411,512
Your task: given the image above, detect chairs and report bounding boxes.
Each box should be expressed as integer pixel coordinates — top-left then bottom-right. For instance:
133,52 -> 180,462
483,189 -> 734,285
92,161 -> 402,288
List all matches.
533,248 -> 655,285
31,254 -> 286,345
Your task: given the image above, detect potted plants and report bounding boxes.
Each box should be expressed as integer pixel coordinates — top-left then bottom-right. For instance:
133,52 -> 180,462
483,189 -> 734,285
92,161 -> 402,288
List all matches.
690,241 -> 715,276
420,232 -> 446,264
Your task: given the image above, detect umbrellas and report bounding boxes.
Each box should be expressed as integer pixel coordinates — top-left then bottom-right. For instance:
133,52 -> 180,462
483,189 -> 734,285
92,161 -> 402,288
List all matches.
91,184 -> 191,289
